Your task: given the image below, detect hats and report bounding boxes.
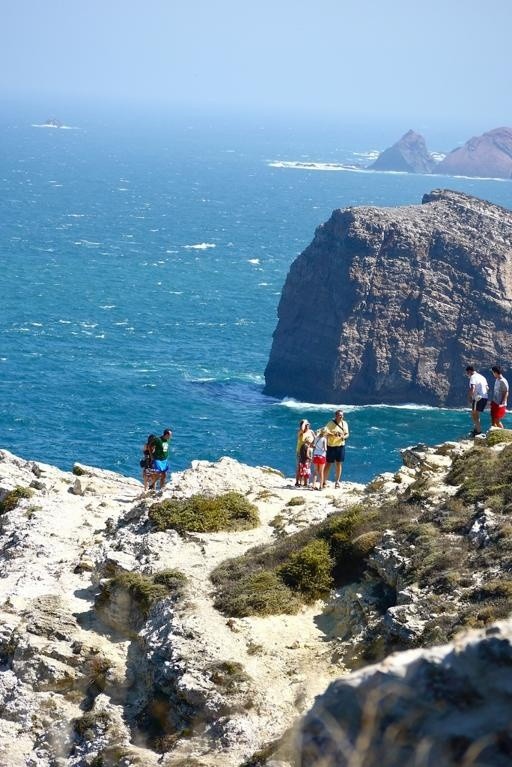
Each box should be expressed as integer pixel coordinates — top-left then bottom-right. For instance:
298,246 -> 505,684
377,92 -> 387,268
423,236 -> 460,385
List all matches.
319,426 -> 329,435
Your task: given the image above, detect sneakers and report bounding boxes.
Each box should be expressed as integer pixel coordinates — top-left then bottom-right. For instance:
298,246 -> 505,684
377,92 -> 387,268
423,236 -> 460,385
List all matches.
469,429 -> 482,438
296,483 -> 340,491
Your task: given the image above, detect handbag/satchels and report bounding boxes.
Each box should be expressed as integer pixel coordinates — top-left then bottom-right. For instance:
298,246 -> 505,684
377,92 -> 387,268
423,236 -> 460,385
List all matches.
153,459 -> 168,472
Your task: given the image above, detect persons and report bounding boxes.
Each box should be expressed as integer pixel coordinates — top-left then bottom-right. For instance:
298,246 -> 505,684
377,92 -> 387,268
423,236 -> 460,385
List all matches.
491,365 -> 510,429
142,434 -> 157,491
147,428 -> 174,489
465,365 -> 489,437
310,426 -> 328,491
295,417 -> 316,488
322,409 -> 350,490
299,435 -> 315,487
